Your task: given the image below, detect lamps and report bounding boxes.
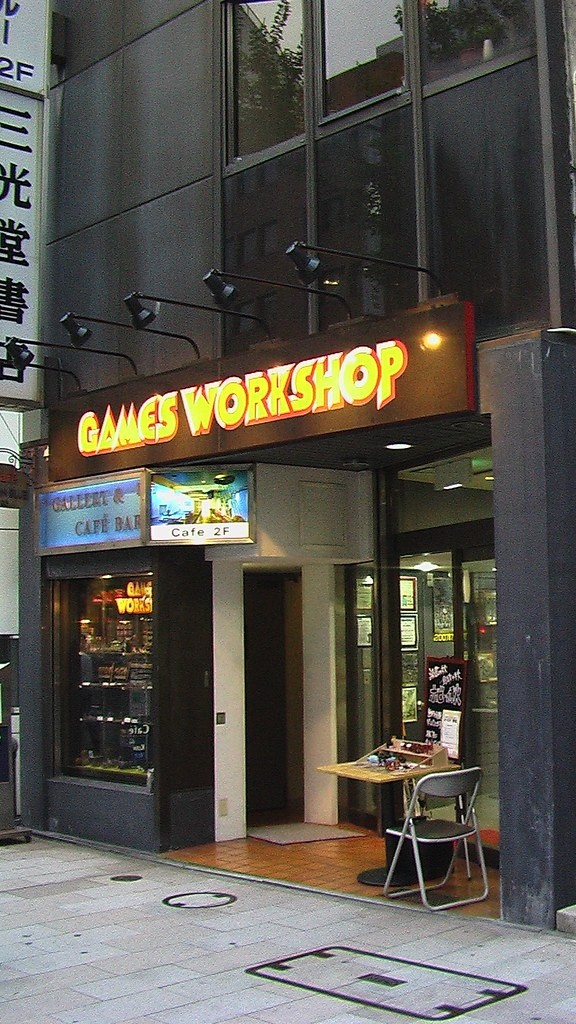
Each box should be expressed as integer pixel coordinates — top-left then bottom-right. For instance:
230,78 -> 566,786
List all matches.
203,267 -> 351,319
59,312 -> 202,358
286,241 -> 442,294
4,336 -> 137,374
123,291 -> 272,338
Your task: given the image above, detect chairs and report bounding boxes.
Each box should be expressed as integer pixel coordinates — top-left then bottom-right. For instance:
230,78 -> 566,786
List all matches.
383,766 -> 490,912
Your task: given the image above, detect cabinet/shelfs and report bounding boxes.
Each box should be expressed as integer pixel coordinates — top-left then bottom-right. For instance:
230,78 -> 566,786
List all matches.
469,570 -> 499,714
68,646 -> 151,785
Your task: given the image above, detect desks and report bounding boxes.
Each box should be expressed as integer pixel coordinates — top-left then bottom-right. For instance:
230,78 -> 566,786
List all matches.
319,761 -> 462,886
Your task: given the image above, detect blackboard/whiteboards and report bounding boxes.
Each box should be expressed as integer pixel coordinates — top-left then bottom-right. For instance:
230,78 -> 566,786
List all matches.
418,655 -> 470,761
